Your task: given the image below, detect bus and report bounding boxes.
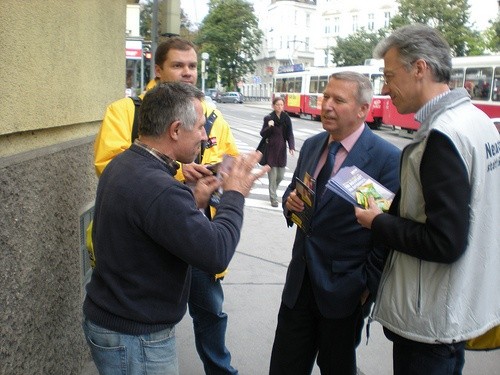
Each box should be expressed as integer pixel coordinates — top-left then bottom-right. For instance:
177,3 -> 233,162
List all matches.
365,54 -> 500,138
270,64 -> 383,131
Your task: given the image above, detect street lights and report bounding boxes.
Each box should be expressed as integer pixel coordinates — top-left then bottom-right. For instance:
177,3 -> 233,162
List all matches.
200,51 -> 210,94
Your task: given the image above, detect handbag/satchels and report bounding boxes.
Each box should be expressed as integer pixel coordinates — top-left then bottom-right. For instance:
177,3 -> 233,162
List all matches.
255,136 -> 269,166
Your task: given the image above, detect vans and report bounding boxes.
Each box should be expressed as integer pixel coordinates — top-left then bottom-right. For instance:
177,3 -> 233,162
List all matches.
205,88 -> 244,104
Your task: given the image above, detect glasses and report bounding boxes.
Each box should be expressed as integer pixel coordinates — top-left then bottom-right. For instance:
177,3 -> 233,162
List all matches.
382,58 -> 418,85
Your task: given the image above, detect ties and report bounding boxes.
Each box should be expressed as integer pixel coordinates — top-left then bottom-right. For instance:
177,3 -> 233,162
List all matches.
315,140 -> 342,198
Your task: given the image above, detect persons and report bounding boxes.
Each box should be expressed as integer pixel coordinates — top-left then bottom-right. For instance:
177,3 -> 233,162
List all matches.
93,38 -> 239,375
82,81 -> 270,375
260,97 -> 295,207
355,20 -> 500,375
268,71 -> 402,375
